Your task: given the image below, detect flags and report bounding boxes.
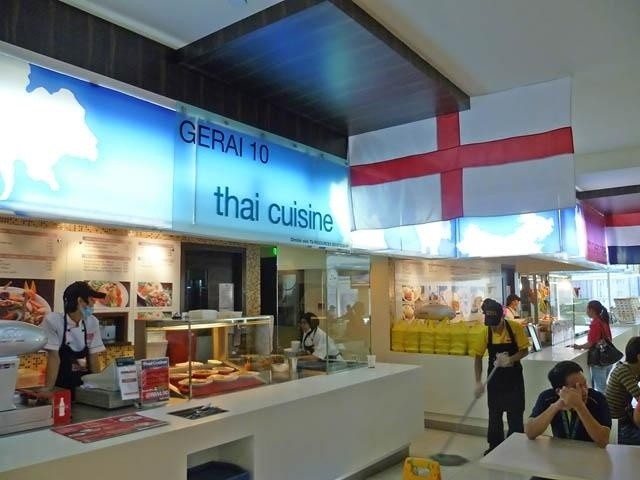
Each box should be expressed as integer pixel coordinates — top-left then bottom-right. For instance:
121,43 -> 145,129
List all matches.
345,77 -> 578,232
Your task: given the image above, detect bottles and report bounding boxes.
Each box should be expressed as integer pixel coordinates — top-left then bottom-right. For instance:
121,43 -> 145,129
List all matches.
59,397 -> 66,417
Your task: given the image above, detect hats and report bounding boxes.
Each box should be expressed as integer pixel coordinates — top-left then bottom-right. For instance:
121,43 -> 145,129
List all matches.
481,299 -> 496,314
507,294 -> 520,305
63,281 -> 107,301
484,303 -> 503,326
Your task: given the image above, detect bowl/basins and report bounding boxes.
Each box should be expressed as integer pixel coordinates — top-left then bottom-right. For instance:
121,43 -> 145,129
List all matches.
272,372 -> 289,380
272,363 -> 289,372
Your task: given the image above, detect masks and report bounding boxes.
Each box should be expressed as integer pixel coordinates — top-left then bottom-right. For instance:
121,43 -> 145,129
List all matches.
80,305 -> 93,320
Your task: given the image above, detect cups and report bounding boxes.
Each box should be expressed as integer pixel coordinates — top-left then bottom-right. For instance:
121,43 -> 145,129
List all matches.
289,357 -> 298,373
368,355 -> 376,368
289,372 -> 298,381
291,340 -> 301,352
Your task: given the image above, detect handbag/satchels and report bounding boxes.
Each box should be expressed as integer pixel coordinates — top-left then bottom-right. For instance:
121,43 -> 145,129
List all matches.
588,336 -> 624,367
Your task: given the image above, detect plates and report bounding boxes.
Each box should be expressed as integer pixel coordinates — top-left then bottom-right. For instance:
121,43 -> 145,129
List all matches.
229,371 -> 260,379
169,373 -> 193,379
402,304 -> 415,321
177,378 -> 213,388
402,286 -> 414,301
176,360 -> 203,369
118,416 -> 142,422
444,289 -> 460,313
206,374 -> 239,382
0,286 -> 52,327
137,282 -> 163,300
212,367 -> 234,374
88,280 -> 129,308
187,369 -> 219,377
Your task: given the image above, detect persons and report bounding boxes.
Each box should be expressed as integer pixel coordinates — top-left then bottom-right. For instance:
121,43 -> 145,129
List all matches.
525,360 -> 612,449
295,311 -> 344,362
327,304 -> 336,326
605,336 -> 640,445
337,304 -> 352,319
633,395 -> 640,429
573,299 -> 614,395
40,281 -> 108,388
345,301 -> 367,337
473,300 -> 533,458
504,293 -> 521,320
